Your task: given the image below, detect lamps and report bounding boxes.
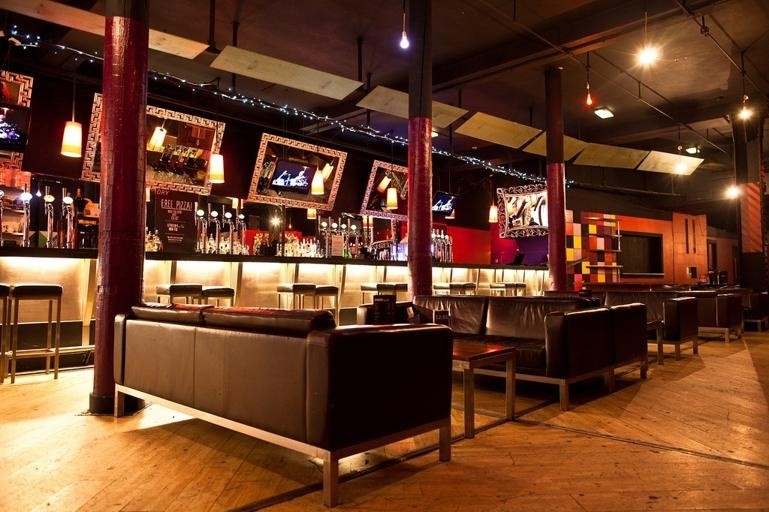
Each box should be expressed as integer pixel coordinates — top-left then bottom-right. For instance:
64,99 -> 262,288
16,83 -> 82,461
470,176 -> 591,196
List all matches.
377,175 -> 391,192
594,108 -> 614,119
399,7 -> 410,51
307,208 -> 317,220
585,65 -> 592,105
210,76 -> 224,184
322,158 -> 337,183
445,160 -> 456,220
61,64 -> 83,159
149,119 -> 167,151
376,142 -> 397,209
310,118 -> 323,196
233,198 -> 244,210
489,171 -> 499,223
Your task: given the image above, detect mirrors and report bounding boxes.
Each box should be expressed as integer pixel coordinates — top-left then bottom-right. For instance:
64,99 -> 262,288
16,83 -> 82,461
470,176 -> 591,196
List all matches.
246,132 -> 347,212
496,183 -> 548,240
81,92 -> 225,197
0,68 -> 33,171
360,159 -> 408,220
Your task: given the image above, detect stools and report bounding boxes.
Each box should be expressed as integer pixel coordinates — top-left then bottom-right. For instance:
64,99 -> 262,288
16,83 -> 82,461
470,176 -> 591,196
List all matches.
0,283 -> 63,384
490,283 -> 527,298
156,283 -> 234,307
432,282 -> 474,298
360,282 -> 408,304
277,283 -> 339,329
